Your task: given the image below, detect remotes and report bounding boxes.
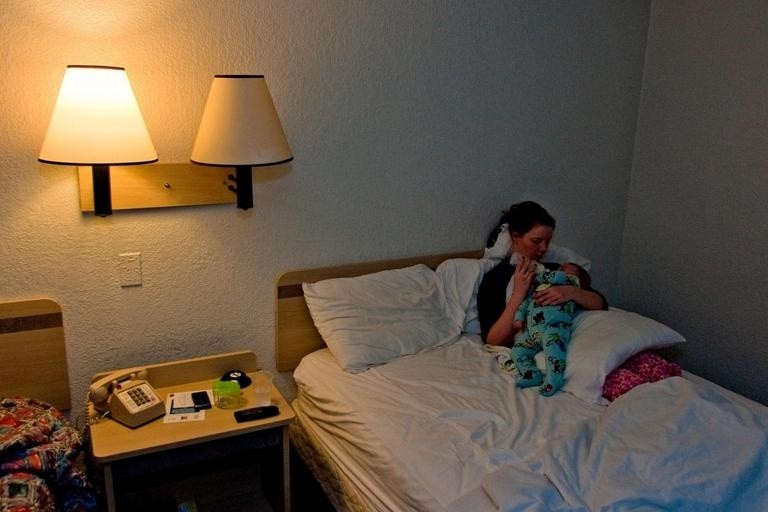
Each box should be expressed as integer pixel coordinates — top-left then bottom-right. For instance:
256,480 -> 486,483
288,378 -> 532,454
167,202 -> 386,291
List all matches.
235,406 -> 282,422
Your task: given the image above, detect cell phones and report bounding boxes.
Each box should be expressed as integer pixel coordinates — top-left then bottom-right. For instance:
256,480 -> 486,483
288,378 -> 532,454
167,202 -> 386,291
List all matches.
192,391 -> 210,409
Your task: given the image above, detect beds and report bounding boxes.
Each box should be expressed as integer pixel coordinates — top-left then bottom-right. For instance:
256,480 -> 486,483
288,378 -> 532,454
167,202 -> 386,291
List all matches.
274,247 -> 768,512
0,298 -> 87,511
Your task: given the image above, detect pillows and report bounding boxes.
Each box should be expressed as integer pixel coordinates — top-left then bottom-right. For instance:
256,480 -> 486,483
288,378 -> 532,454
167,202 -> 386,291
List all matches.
436,257 -> 503,350
530,300 -> 683,407
494,231 -> 594,277
301,260 -> 467,374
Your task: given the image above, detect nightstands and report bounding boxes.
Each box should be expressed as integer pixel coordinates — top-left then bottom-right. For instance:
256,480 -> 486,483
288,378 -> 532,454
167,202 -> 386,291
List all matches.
87,351 -> 296,512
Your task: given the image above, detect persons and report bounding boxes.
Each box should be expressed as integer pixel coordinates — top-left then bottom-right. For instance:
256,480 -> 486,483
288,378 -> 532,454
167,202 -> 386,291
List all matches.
511,262 -> 591,396
478,200 -> 682,402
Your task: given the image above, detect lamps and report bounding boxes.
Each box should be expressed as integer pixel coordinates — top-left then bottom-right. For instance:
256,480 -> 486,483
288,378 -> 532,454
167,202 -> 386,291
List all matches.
38,65 -> 294,217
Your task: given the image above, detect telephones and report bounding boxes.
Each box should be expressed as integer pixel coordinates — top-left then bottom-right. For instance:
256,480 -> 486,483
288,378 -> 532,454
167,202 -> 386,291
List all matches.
89,366 -> 167,429
